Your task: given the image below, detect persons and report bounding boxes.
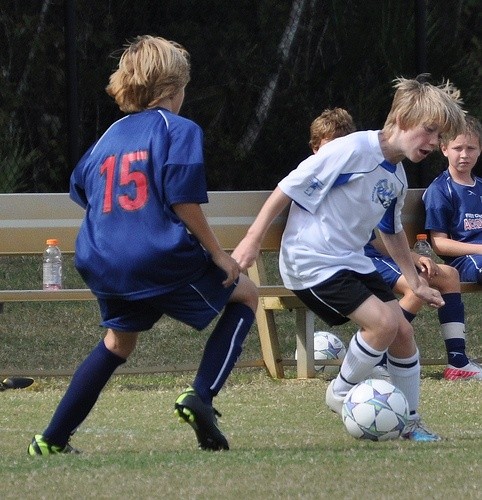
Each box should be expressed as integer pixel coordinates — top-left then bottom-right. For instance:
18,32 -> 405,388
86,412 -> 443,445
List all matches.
27,36 -> 260,457
310,108 -> 482,380
231,74 -> 471,441
423,115 -> 482,284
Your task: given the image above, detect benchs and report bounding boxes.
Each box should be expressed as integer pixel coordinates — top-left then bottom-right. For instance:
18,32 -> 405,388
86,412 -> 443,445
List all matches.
0,188 -> 482,379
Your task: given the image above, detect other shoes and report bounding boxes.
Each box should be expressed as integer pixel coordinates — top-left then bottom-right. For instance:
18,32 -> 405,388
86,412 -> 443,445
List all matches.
27,435 -> 81,460
398,419 -> 447,441
443,362 -> 482,381
326,380 -> 346,417
371,365 -> 390,379
174,387 -> 230,451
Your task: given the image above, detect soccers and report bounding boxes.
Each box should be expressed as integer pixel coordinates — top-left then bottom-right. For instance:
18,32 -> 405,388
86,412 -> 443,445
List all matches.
295,331 -> 346,373
341,379 -> 409,442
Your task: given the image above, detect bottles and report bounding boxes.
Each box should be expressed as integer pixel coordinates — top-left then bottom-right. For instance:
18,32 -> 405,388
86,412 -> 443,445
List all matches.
414,234 -> 433,258
43,239 -> 62,289
